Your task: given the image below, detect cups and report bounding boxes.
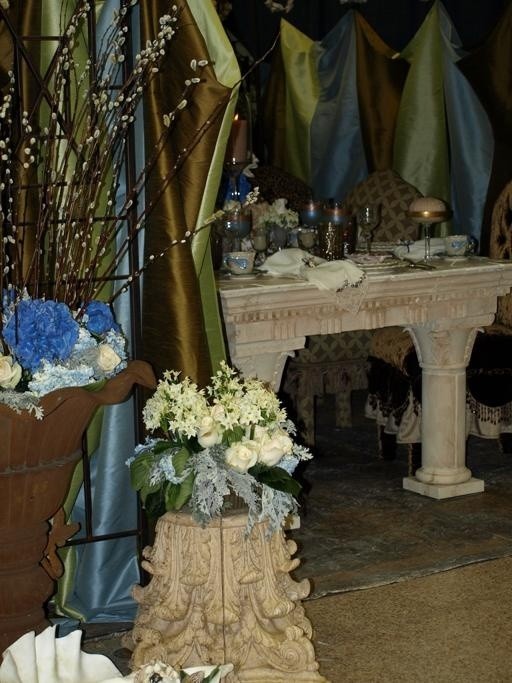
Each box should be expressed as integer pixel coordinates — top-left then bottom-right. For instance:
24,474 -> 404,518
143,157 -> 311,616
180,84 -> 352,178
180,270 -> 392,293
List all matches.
442,235 -> 475,256
223,251 -> 256,275
301,200 -> 350,227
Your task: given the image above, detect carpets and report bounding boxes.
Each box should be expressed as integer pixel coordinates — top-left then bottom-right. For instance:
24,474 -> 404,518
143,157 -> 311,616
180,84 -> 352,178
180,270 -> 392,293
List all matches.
287,401 -> 511,602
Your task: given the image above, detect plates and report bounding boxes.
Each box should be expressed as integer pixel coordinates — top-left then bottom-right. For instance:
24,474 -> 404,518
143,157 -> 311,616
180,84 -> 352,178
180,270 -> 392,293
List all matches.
344,253 -> 411,274
224,269 -> 260,280
432,251 -> 472,263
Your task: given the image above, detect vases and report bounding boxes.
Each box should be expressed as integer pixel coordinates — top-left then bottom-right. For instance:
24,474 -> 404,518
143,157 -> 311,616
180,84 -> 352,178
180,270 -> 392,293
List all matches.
1,360 -> 158,665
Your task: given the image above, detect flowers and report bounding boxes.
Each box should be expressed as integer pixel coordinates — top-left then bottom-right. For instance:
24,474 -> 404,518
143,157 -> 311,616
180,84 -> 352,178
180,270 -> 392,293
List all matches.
1,284 -> 124,420
125,360 -> 314,540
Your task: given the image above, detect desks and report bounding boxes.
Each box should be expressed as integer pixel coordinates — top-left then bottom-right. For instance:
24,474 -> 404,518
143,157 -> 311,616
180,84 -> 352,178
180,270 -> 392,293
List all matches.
214,257 -> 511,517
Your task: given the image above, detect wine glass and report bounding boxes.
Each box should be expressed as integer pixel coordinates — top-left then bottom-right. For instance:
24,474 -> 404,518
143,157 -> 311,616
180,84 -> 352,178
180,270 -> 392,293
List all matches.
223,207 -> 251,251
358,205 -> 381,252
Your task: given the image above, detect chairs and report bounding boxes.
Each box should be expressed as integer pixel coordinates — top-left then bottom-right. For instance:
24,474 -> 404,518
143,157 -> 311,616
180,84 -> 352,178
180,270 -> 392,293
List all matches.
399,170 -> 512,449
342,162 -> 438,438
258,165 -> 328,244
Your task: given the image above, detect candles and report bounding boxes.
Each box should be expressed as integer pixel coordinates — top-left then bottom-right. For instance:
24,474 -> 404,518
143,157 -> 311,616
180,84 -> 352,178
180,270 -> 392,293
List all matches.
223,113 -> 248,164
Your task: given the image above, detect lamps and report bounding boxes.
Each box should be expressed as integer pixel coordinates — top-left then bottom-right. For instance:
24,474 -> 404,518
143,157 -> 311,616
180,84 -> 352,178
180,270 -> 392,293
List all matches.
409,196 -> 448,269
